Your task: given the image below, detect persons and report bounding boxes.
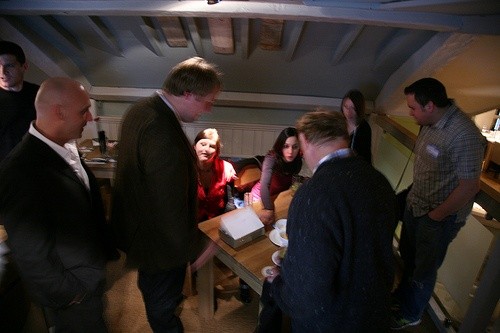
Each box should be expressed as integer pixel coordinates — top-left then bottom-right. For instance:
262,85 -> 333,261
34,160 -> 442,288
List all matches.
0,41 -> 40,161
0,77 -> 108,333
190,128 -> 238,295
111,56 -> 221,333
235,127 -> 305,303
255,110 -> 397,333
340,89 -> 373,168
389,78 -> 486,329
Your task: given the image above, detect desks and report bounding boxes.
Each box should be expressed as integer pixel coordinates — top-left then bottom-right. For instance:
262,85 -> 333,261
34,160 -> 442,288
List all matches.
195,206 -> 278,318
77,138 -> 119,188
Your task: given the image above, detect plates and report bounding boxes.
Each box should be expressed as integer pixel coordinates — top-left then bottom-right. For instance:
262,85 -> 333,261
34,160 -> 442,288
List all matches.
269,229 -> 289,247
272,250 -> 282,267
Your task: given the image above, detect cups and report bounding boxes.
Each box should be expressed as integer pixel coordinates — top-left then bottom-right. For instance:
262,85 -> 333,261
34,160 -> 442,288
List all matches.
275,219 -> 289,240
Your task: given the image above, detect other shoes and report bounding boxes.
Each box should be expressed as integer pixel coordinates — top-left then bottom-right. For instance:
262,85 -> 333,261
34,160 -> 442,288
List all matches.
239,280 -> 252,303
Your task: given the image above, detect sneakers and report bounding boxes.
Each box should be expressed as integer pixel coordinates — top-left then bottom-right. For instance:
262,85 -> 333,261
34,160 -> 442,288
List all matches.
390,309 -> 421,329
389,294 -> 400,306
